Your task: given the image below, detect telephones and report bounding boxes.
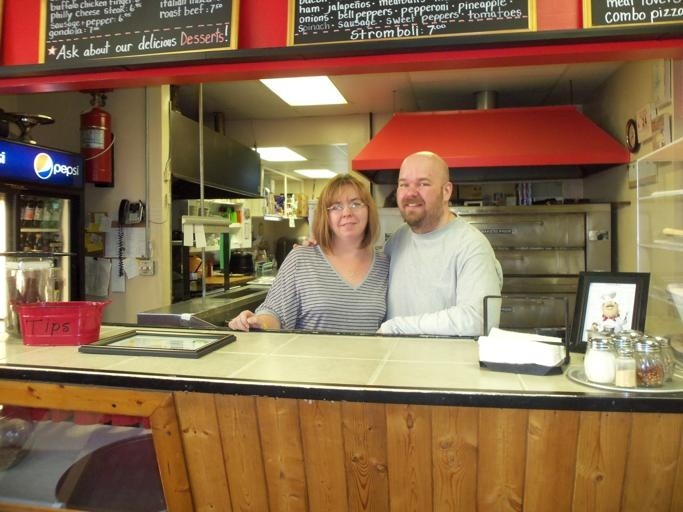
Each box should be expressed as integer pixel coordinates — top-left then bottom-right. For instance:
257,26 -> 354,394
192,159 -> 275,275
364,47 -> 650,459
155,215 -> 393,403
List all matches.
118,199 -> 144,225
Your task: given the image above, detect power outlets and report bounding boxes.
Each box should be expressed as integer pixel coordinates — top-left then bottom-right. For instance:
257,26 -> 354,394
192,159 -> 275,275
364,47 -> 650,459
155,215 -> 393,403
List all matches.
140,260 -> 155,276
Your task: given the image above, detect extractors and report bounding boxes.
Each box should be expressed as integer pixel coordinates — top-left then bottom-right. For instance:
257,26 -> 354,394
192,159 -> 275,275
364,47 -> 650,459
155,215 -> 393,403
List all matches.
351,104 -> 631,185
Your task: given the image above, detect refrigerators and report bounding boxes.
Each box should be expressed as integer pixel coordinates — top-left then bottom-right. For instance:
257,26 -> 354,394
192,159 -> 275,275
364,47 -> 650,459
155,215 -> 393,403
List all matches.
1,136 -> 85,343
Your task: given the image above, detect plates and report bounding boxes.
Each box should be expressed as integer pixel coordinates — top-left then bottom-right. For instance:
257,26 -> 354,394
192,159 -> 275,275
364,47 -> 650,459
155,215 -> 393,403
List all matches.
566,366 -> 683,394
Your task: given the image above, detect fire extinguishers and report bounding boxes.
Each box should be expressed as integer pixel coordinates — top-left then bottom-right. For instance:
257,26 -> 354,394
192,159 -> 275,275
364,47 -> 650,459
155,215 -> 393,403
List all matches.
79,92 -> 113,184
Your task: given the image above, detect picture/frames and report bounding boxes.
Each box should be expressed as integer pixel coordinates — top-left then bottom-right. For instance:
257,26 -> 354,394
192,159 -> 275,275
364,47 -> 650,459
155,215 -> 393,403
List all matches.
78,330 -> 236,359
570,271 -> 649,353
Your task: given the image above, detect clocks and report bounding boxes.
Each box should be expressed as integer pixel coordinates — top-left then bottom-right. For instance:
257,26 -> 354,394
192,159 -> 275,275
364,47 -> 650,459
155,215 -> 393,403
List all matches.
626,119 -> 639,152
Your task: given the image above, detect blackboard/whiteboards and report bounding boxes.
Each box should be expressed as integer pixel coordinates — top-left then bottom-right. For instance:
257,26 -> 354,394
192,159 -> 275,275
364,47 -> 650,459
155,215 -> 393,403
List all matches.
583,0 -> 683,29
39,0 -> 239,64
287,0 -> 537,46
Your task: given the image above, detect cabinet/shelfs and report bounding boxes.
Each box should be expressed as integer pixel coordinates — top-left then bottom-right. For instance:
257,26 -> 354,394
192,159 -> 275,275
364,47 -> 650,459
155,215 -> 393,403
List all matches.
0,183 -> 70,336
0,326 -> 683,512
261,167 -> 306,219
637,138 -> 683,379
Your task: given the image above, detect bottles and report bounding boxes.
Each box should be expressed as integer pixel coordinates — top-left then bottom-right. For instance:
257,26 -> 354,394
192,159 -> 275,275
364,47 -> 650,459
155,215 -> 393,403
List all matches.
657,336 -> 676,380
54,282 -> 61,302
24,198 -> 33,228
594,329 -> 653,345
19,232 -> 62,252
615,336 -> 636,387
41,201 -> 49,228
635,340 -> 666,386
18,194 -> 25,227
585,338 -> 618,384
50,200 -> 61,228
34,200 -> 42,227
22,272 -> 42,303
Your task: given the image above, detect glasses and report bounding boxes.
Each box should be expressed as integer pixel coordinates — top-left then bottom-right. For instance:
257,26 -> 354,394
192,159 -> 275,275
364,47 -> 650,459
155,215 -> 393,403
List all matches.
327,201 -> 366,212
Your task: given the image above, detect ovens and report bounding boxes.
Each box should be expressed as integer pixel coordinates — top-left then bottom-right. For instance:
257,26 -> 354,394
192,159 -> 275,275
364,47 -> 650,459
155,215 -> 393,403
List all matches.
307,199 -> 612,337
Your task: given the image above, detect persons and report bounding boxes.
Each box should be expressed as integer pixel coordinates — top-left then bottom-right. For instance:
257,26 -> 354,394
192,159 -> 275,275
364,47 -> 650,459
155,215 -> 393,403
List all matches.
228,174 -> 389,332
380,151 -> 503,336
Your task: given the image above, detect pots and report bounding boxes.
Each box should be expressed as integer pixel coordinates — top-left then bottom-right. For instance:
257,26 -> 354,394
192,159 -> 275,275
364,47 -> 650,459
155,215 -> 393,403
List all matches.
230,250 -> 254,276
